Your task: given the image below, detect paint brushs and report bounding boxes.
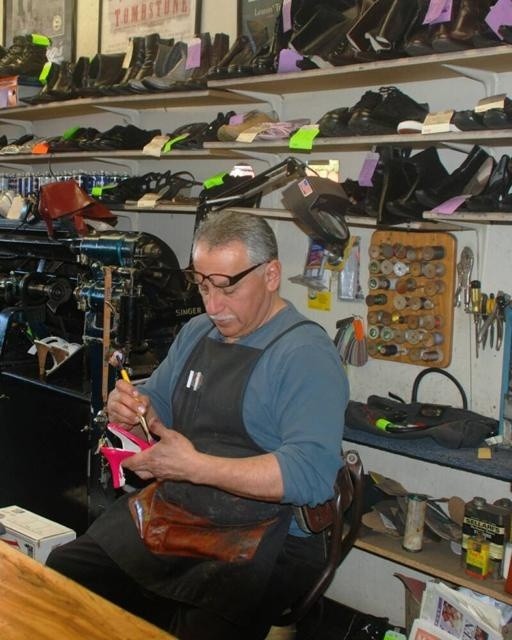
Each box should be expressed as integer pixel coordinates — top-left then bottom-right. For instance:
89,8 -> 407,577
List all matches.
116,354 -> 155,446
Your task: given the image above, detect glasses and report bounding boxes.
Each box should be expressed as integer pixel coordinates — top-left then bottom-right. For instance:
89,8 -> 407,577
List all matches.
182,260 -> 272,288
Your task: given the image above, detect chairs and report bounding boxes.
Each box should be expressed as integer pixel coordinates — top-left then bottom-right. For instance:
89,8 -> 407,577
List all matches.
267,443 -> 366,626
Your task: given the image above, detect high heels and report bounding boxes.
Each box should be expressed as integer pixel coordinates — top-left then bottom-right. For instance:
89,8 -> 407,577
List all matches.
35,178 -> 118,236
91,170 -> 203,205
27,336 -> 82,377
100,422 -> 152,490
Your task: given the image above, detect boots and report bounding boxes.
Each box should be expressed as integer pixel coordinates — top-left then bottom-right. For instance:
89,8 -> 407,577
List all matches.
377,144 -> 511,227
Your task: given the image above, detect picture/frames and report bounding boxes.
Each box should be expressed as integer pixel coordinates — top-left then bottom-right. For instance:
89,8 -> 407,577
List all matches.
95,1 -> 204,59
236,1 -> 283,40
1,0 -> 79,68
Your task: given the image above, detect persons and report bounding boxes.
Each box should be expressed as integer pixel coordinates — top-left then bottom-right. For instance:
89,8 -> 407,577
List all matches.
42,205 -> 352,639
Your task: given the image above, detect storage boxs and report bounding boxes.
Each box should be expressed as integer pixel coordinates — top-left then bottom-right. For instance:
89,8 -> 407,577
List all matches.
0,503 -> 78,565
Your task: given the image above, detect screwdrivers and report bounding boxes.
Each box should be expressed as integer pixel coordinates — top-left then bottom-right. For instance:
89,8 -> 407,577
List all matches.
471,281 -> 481,357
482,294 -> 488,350
490,293 -> 495,350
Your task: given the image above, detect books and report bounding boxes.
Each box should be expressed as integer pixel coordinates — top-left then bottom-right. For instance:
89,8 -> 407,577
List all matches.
409,580 -> 512,639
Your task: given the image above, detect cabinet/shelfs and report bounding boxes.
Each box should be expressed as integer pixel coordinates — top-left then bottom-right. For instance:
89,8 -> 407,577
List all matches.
336,421 -> 512,611
1,45 -> 512,233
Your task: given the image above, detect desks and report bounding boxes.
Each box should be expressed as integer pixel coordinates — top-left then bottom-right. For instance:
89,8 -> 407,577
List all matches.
0,542 -> 178,640
